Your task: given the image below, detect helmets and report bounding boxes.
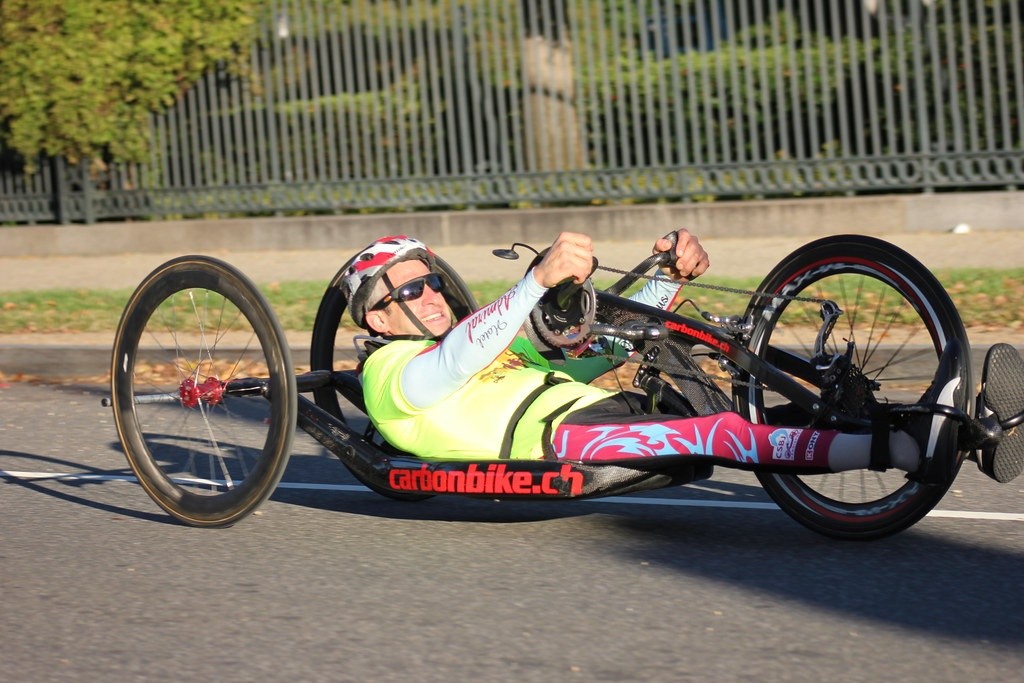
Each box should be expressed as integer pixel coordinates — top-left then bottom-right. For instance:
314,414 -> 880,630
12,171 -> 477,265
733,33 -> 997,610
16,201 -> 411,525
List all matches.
340,235 -> 436,329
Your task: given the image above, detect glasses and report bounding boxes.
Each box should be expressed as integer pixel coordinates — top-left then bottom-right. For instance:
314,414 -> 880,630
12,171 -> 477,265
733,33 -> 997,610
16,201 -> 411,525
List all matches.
369,273 -> 445,311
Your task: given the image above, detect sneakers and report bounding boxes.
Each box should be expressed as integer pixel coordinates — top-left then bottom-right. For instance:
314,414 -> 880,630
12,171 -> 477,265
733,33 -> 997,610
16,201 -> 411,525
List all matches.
967,343 -> 1024,483
892,338 -> 967,489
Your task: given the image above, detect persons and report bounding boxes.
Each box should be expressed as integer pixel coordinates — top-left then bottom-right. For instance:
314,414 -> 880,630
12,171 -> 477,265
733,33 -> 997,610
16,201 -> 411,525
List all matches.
339,228 -> 1024,484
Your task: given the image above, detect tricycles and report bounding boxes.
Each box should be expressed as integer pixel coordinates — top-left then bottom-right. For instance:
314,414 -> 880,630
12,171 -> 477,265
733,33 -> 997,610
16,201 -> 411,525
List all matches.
100,235 -> 1024,542
